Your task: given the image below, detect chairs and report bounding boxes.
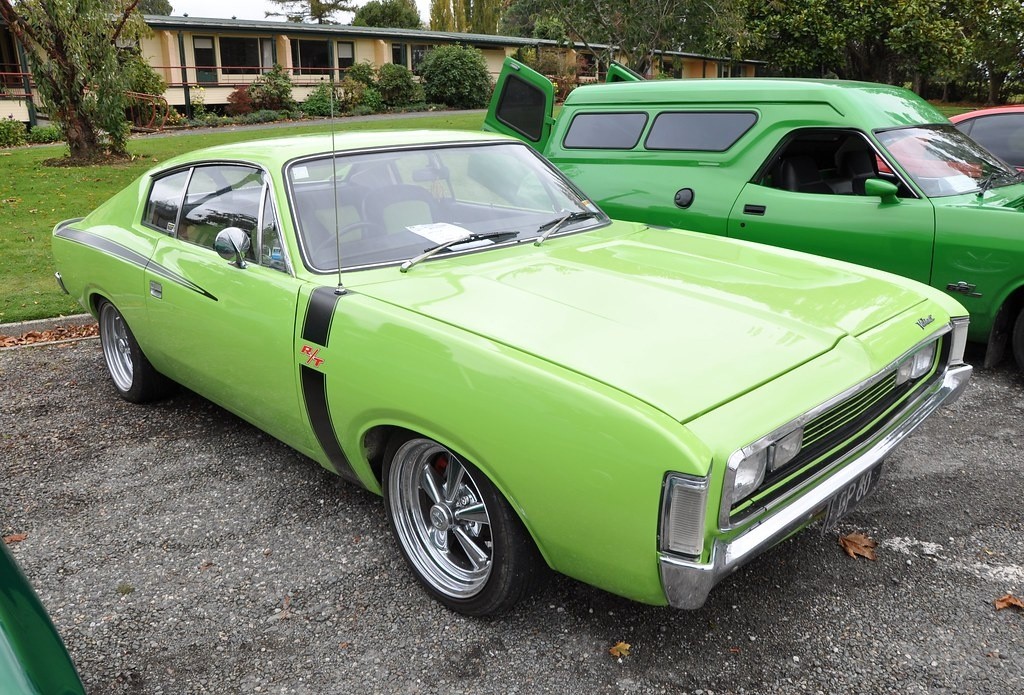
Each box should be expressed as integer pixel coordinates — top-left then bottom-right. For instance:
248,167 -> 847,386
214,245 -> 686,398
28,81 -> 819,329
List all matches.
781,154 -> 838,196
363,184 -> 447,235
299,192 -> 369,252
837,150 -> 877,198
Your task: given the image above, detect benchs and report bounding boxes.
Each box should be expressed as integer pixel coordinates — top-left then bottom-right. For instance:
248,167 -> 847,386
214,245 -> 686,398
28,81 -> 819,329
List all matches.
183,186 -> 350,246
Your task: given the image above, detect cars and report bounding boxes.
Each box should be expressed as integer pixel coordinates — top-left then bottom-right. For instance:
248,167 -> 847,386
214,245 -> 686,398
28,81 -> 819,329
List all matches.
873,104 -> 1024,175
52,126 -> 976,620
483,57 -> 1024,373
